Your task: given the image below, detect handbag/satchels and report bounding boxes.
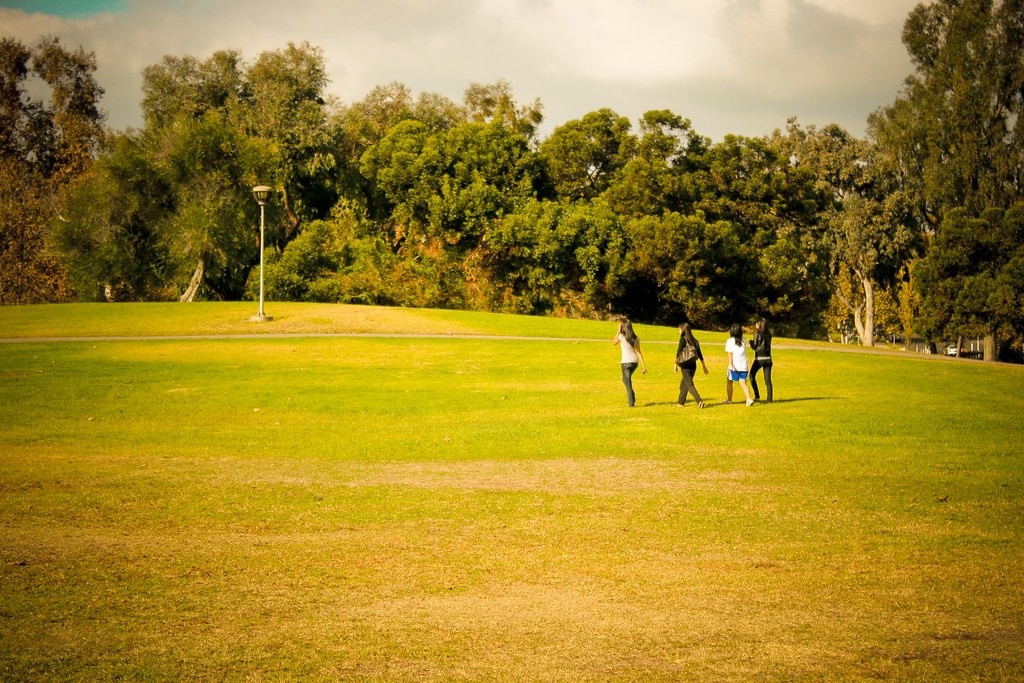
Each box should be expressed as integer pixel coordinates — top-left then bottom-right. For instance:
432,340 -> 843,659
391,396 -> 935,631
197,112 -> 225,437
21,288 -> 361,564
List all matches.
674,336 -> 698,366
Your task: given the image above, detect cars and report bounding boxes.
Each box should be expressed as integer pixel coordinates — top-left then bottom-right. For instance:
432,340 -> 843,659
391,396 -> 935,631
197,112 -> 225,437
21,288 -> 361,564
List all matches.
948,345 -> 958,356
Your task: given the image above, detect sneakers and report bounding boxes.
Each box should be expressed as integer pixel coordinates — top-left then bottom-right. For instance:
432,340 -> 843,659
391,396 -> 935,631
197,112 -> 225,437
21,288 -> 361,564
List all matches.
746,399 -> 754,406
722,400 -> 733,404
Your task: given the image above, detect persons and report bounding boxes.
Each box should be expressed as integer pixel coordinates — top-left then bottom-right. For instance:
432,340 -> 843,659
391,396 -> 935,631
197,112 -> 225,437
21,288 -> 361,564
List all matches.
723,323 -> 754,406
612,319 -> 646,407
673,322 -> 709,409
749,317 -> 773,404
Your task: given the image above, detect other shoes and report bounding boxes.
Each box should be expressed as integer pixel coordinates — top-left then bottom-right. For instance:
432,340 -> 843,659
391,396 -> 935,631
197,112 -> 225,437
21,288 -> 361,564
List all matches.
627,403 -> 634,407
677,403 -> 684,408
699,401 -> 705,408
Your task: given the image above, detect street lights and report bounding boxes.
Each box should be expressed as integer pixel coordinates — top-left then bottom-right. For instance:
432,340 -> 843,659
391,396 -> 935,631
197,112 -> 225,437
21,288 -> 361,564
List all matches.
252,185 -> 273,323
